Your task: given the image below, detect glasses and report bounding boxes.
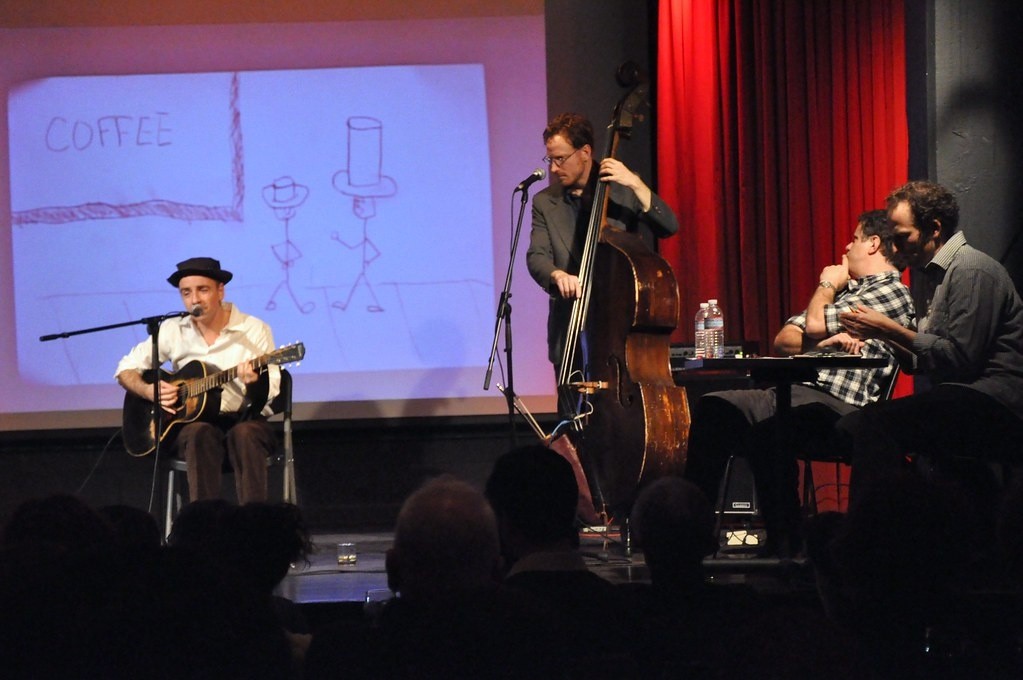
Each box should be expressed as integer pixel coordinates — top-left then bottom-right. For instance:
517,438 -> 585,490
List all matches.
541,145 -> 584,168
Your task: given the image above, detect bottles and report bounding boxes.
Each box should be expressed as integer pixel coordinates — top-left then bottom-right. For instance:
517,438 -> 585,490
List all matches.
704,300 -> 724,359
695,303 -> 710,357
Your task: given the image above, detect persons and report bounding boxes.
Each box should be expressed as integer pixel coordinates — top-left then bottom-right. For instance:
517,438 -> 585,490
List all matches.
629,480 -> 718,590
315,471 -> 510,679
837,180 -> 1023,541
118,256 -> 287,508
528,115 -> 677,522
695,212 -> 915,558
485,447 -> 630,623
154,496 -> 316,680
1,481 -> 163,680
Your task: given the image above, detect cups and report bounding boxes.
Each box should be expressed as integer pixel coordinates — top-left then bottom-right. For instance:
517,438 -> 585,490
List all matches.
337,542 -> 356,567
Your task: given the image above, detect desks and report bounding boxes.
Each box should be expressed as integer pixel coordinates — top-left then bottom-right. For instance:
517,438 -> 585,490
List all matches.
672,355 -> 889,561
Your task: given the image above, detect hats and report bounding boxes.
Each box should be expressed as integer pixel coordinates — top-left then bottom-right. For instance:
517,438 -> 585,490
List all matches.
167,257 -> 232,287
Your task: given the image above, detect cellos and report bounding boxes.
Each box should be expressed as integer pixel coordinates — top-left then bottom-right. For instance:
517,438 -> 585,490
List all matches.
557,59 -> 692,562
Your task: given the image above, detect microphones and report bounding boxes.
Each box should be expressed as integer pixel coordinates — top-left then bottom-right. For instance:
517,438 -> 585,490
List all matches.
514,168 -> 546,193
191,305 -> 204,317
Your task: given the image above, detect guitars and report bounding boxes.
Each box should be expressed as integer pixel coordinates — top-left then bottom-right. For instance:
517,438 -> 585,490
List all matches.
122,340 -> 306,458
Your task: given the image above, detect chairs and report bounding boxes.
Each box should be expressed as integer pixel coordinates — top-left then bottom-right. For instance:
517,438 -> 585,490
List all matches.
793,360 -> 901,558
167,369 -> 295,544
